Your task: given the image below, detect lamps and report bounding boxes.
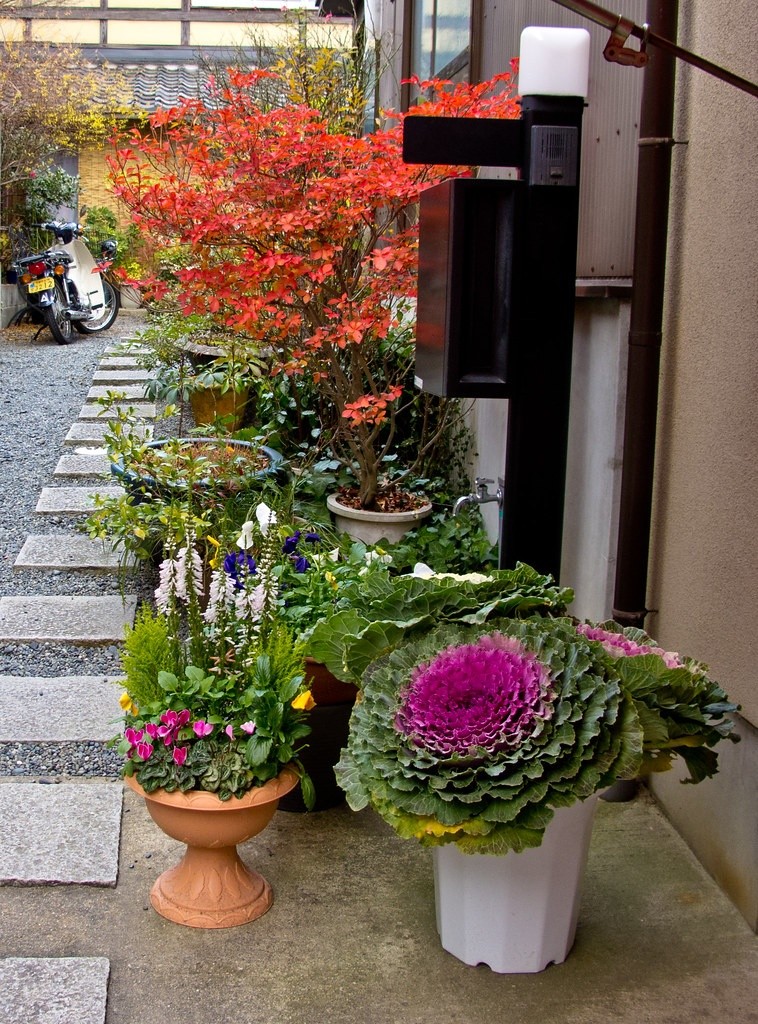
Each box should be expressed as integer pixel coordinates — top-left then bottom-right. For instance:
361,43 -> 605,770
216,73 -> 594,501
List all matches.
516,26 -> 592,112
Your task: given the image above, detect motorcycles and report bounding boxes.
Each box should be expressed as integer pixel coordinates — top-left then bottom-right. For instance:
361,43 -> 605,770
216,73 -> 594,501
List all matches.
12,220 -> 119,345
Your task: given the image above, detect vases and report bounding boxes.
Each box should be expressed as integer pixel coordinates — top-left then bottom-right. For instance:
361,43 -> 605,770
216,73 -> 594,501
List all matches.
119,286 -> 141,310
276,657 -> 360,815
6,269 -> 18,284
122,761 -> 300,930
432,779 -> 614,974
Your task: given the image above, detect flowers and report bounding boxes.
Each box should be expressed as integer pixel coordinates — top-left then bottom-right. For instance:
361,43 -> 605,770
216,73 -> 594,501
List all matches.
333,619 -> 745,855
106,474 -> 317,815
203,497 -> 393,634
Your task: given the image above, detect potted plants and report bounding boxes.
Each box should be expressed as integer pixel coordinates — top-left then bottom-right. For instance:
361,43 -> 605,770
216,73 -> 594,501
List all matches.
68,34 -> 522,549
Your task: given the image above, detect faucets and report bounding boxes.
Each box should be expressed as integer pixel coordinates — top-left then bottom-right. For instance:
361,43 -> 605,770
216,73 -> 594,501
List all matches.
452,476 -> 502,517
